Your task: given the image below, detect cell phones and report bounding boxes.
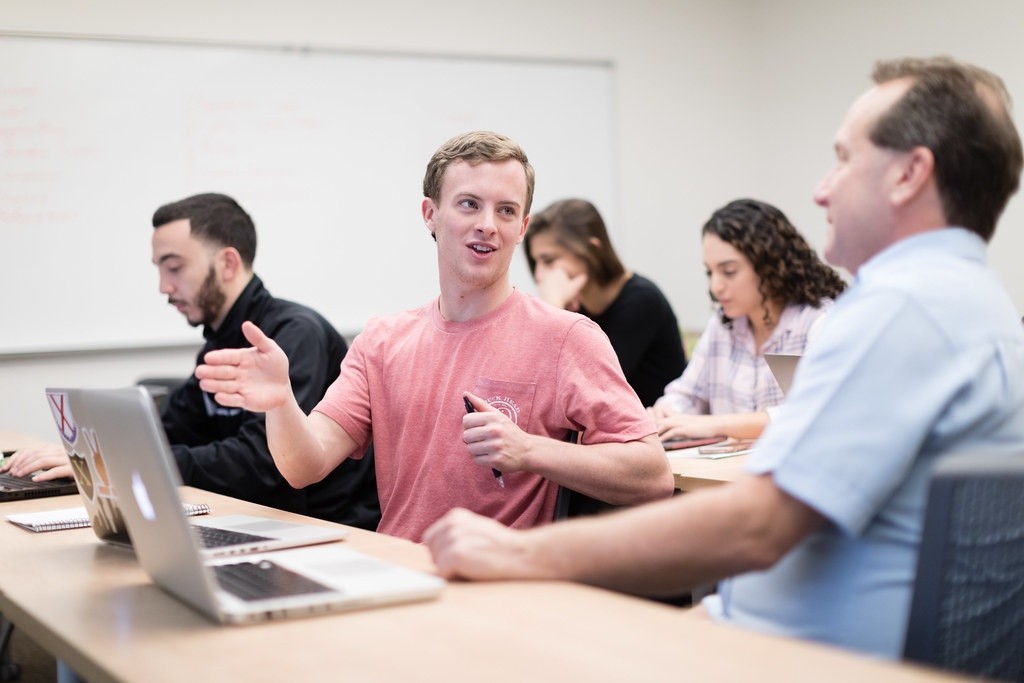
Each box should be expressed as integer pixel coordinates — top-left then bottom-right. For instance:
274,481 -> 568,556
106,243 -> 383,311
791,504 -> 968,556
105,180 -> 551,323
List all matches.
698,441 -> 752,454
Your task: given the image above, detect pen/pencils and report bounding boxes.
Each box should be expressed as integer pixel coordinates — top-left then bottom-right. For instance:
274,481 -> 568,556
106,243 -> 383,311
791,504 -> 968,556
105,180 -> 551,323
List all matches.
463,394 -> 505,489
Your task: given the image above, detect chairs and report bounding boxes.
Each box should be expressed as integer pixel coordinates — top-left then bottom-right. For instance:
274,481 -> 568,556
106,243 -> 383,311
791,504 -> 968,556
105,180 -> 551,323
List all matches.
904,445 -> 1024,683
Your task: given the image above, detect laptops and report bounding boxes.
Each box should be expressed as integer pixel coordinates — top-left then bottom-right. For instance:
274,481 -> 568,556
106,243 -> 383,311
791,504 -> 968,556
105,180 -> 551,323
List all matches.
0,386 -> 444,623
662,436 -> 728,450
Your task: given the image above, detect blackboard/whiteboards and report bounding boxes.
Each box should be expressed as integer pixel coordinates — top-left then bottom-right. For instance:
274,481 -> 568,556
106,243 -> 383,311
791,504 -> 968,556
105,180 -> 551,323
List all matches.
2,27 -> 624,363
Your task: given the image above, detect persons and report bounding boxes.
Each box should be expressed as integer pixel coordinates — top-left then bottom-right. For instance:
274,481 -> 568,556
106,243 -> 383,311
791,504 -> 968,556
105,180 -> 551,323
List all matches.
524,198 -> 688,409
195,130 -> 674,544
0,194 -> 365,683
423,56 -> 1024,659
645,195 -> 847,598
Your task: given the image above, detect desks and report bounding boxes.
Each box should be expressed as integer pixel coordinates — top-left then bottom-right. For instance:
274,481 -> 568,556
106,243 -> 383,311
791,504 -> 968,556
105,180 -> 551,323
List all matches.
667,435 -> 757,607
0,433 -> 988,683
